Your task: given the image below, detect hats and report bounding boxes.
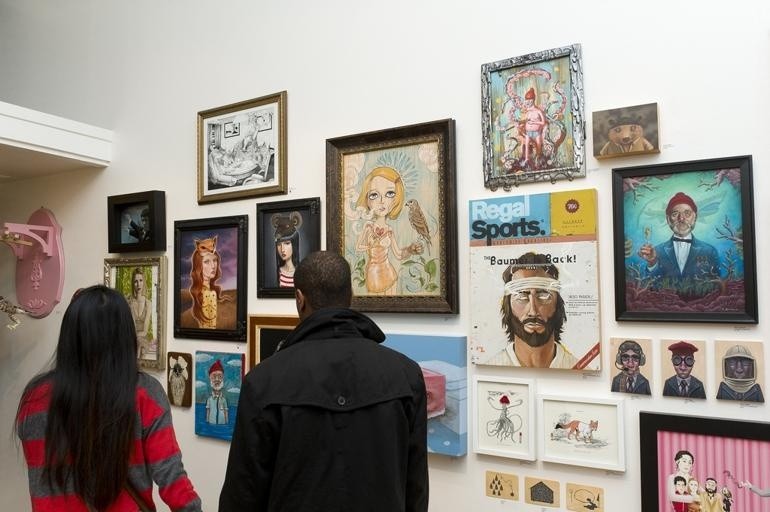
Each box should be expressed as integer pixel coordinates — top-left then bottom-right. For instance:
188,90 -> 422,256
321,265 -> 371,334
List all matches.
666,191 -> 698,215
209,360 -> 226,374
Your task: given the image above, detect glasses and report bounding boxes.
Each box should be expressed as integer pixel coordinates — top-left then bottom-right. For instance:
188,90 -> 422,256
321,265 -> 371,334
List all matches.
726,357 -> 753,368
622,354 -> 640,362
673,353 -> 695,367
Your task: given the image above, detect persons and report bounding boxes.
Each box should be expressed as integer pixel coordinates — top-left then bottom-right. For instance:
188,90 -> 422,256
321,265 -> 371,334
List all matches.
671,476 -> 688,512
206,359 -> 230,426
610,341 -> 651,396
273,213 -> 300,288
667,451 -> 720,512
699,479 -> 724,512
511,89 -> 544,169
354,166 -> 417,296
128,209 -> 151,246
715,346 -> 766,403
190,234 -> 221,330
661,342 -> 706,399
17,287 -> 204,512
481,253 -> 591,371
637,191 -> 723,298
125,267 -> 151,357
219,252 -> 430,512
685,478 -> 701,512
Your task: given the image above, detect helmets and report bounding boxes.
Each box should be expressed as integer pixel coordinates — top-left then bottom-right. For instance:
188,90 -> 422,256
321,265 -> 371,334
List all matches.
722,344 -> 758,391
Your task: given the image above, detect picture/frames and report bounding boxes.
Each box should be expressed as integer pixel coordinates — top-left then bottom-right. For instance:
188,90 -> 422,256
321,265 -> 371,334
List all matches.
326,118 -> 460,314
481,43 -> 585,192
612,155 -> 758,325
256,197 -> 321,299
639,410 -> 770,512
249,314 -> 300,374
173,214 -> 248,343
107,190 -> 166,253
537,395 -> 626,472
197,90 -> 288,205
472,374 -> 537,462
103,256 -> 168,370
